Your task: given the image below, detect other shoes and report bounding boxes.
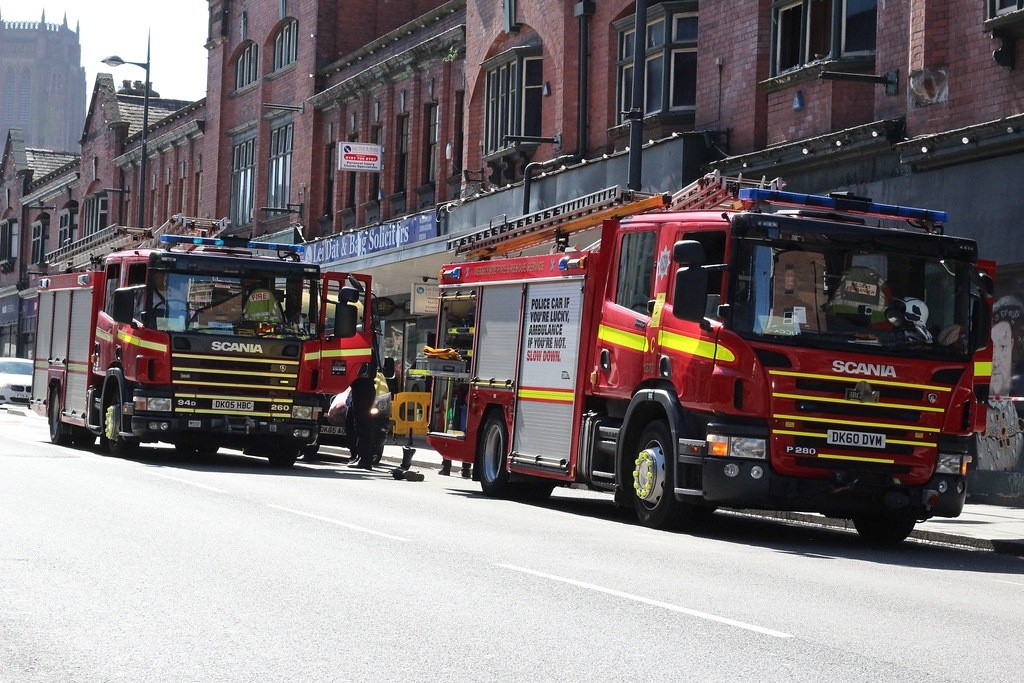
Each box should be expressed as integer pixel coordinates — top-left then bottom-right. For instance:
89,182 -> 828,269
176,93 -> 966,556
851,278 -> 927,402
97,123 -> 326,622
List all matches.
348,456 -> 372,470
438,467 -> 450,476
461,467 -> 470,477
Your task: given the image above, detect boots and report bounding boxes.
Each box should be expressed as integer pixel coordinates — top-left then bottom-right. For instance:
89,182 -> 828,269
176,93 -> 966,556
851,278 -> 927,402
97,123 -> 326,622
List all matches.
396,445 -> 416,470
391,466 -> 425,481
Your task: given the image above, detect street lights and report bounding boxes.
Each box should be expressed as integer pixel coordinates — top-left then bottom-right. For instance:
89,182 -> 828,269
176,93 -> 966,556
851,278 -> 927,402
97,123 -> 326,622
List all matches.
99,26 -> 151,230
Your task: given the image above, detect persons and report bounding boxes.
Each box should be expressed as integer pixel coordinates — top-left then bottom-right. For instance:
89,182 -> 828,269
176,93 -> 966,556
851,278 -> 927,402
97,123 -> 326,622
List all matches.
139,272 -> 188,328
824,267 -> 895,327
438,457 -> 471,479
243,280 -> 286,325
344,358 -> 401,468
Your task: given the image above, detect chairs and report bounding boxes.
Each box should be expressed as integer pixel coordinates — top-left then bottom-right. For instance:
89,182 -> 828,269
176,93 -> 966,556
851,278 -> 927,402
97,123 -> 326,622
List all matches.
242,290 -> 285,324
825,266 -> 897,342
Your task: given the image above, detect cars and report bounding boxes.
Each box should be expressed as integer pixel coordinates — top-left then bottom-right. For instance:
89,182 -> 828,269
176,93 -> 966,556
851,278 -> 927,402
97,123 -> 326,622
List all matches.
0,358 -> 37,405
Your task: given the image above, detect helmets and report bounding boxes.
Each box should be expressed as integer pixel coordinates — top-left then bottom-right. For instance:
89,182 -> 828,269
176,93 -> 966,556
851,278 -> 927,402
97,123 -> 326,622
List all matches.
893,296 -> 930,327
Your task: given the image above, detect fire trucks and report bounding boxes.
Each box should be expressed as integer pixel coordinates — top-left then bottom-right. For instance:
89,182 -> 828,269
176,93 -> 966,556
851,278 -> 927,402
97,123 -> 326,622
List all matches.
29,234 -> 373,469
415,169 -> 998,549
